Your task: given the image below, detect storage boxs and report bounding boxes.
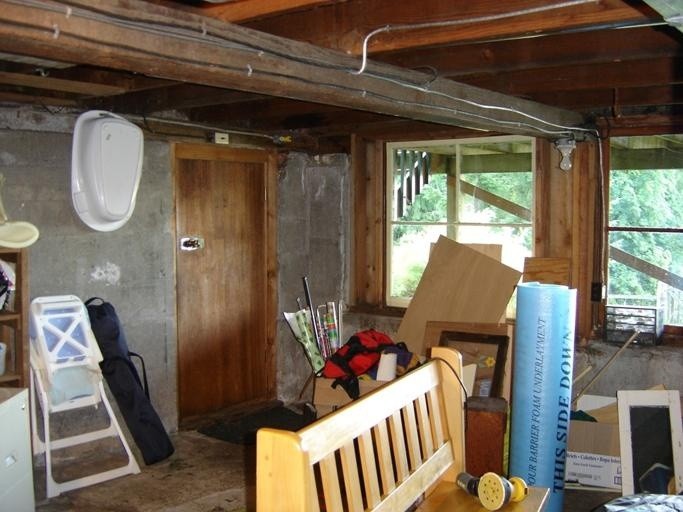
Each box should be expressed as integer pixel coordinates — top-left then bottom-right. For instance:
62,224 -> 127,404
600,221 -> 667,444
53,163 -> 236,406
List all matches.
311,372 -> 390,421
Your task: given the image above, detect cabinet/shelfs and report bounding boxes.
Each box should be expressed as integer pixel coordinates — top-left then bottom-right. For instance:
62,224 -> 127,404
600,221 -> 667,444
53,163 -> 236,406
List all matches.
0,241 -> 32,392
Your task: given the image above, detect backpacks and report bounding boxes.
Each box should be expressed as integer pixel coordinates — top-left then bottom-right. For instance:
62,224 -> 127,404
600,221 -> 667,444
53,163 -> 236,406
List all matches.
322,330 -> 395,380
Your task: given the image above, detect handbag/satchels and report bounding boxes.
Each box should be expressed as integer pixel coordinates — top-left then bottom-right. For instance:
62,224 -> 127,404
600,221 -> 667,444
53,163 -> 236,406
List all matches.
82,294 -> 176,466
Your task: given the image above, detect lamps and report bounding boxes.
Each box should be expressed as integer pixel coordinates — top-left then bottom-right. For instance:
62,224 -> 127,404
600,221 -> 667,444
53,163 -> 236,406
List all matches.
555,137 -> 577,171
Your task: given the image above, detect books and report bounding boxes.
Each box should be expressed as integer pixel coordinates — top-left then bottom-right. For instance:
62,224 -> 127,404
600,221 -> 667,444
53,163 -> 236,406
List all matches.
4,325 -> 15,373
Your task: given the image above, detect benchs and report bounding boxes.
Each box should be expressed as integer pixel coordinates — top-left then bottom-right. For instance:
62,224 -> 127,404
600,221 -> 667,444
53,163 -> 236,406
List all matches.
255,346 -> 552,511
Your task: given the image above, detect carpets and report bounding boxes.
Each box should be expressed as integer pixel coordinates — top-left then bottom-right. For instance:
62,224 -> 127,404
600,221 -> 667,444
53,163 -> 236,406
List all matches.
198,404 -> 314,446
